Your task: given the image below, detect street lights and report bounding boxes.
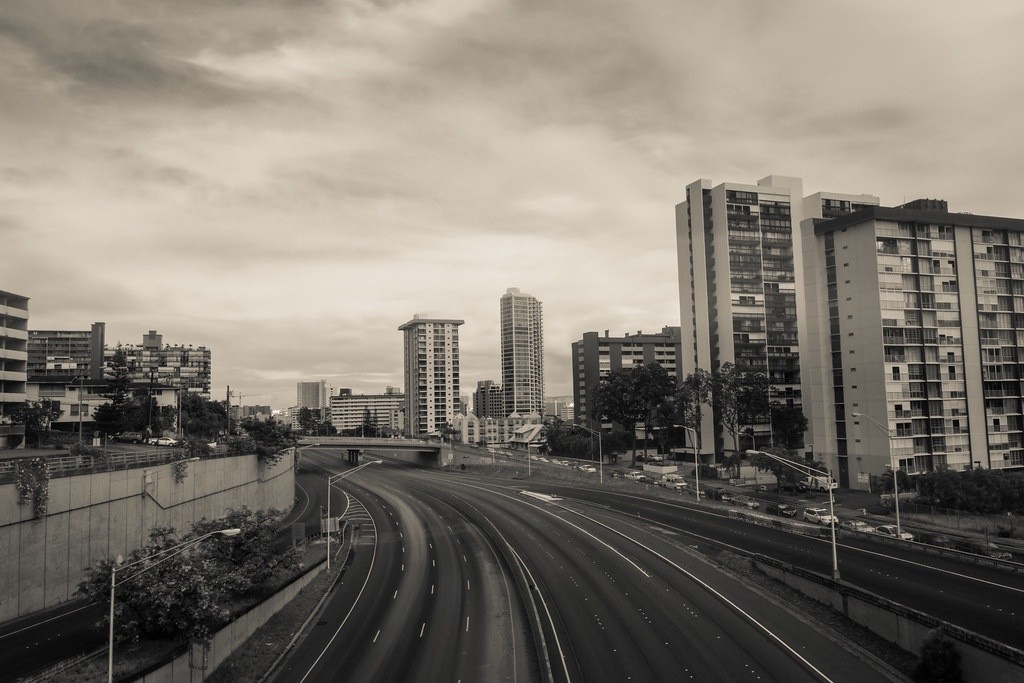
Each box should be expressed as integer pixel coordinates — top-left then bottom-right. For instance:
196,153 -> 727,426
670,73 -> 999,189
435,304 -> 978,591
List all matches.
326,458 -> 383,572
572,423 -> 603,484
745,449 -> 840,579
850,410 -> 902,540
77,375 -> 92,447
673,424 -> 701,501
108,526 -> 242,683
295,443 -> 320,475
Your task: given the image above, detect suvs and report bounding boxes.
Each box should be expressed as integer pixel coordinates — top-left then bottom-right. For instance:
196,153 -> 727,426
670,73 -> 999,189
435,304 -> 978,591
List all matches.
802,507 -> 839,527
112,431 -> 142,444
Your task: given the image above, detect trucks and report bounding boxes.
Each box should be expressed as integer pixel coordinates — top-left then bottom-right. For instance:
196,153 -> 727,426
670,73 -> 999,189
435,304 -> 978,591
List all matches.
800,476 -> 838,493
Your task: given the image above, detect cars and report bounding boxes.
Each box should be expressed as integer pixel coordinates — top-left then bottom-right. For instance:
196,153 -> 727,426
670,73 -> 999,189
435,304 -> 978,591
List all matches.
647,455 -> 663,461
955,534 -> 1013,562
917,532 -> 952,549
636,455 -> 651,462
871,525 -> 915,541
766,503 -> 797,518
206,436 -> 235,451
780,482 -> 808,494
148,437 -> 177,447
468,444 -> 761,509
839,519 -> 874,533
